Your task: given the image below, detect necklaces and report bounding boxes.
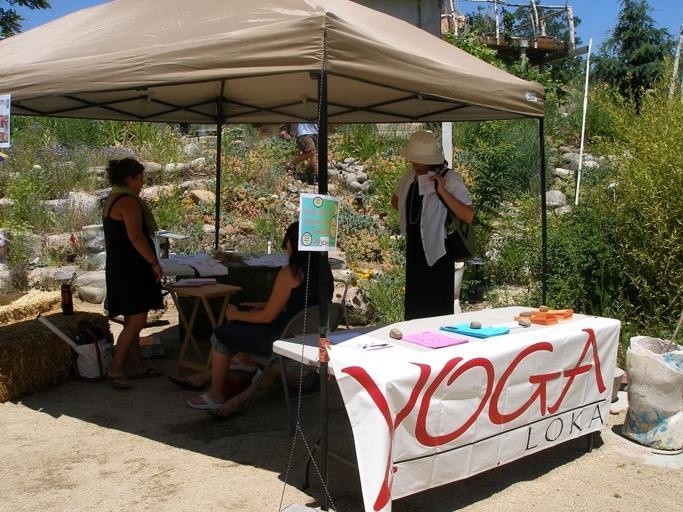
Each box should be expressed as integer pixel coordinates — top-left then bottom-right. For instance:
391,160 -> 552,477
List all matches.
409,174 -> 423,224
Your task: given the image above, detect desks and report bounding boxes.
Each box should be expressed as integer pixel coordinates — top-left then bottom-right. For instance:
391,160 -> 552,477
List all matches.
270,306 -> 625,510
155,249 -> 344,345
159,274 -> 243,381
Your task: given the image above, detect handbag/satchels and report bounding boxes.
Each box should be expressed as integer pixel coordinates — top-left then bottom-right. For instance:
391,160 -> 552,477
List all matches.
444,206 -> 477,263
76,328 -> 113,381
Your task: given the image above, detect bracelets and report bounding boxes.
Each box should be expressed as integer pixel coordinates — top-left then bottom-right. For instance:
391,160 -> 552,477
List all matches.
148,255 -> 159,267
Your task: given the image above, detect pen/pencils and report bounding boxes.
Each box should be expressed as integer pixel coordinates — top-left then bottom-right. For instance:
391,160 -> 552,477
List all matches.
362,343 -> 390,349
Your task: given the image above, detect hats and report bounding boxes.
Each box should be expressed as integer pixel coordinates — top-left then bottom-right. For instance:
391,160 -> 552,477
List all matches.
398,131 -> 445,165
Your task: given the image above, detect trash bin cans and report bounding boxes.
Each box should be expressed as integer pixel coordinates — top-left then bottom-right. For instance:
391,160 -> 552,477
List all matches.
621,335 -> 683,451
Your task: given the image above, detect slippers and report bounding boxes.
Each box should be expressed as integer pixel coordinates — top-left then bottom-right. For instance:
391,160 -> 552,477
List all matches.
111,374 -> 133,390
169,373 -> 204,391
229,356 -> 258,372
130,367 -> 160,379
187,393 -> 241,419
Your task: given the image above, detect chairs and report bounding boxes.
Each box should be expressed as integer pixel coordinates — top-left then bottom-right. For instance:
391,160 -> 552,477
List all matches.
234,298 -> 344,442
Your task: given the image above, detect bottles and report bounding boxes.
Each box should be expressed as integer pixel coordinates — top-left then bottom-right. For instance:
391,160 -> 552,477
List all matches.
60,283 -> 73,314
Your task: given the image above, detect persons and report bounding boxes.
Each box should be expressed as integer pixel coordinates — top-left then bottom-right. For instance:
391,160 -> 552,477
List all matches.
101,156 -> 163,388
286,123 -> 319,181
390,130 -> 474,322
186,220 -> 333,410
169,350 -> 309,420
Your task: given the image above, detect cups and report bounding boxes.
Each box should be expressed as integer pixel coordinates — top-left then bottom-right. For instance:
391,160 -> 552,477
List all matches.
160,237 -> 170,258
